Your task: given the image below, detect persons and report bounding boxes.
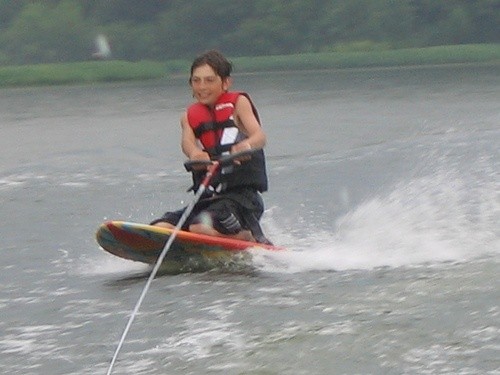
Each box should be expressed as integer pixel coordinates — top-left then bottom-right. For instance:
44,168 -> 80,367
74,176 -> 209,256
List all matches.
148,48 -> 269,242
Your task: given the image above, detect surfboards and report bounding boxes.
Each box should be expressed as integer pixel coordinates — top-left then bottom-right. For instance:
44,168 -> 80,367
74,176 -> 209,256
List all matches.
97,220 -> 286,265
